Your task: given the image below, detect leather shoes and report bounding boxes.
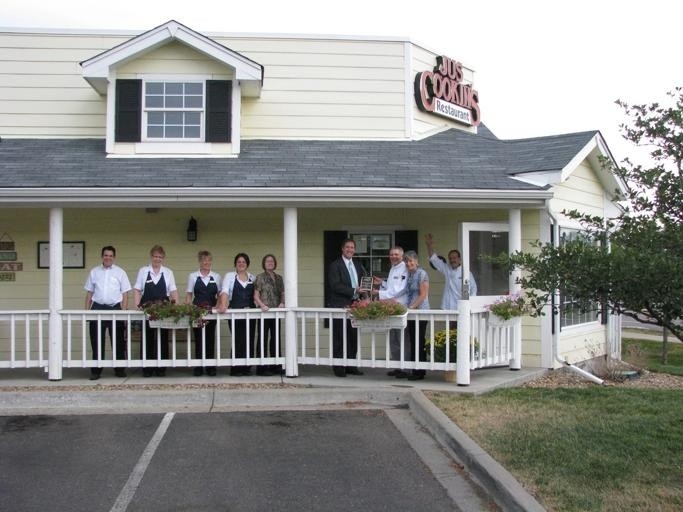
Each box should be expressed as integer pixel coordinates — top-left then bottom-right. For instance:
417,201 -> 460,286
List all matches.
386,368 -> 426,380
88,373 -> 100,380
113,370 -> 127,378
269,365 -> 285,374
206,369 -> 216,376
154,370 -> 165,377
229,369 -> 253,376
192,368 -> 204,376
346,367 -> 364,376
332,366 -> 345,378
143,372 -> 152,377
255,367 -> 273,377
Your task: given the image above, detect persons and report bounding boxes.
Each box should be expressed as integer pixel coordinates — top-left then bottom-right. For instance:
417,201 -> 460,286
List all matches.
403,249 -> 430,380
370,246 -> 410,378
254,254 -> 284,376
328,237 -> 373,377
219,253 -> 257,376
133,245 -> 179,376
185,251 -> 223,376
83,247 -> 132,380
426,232 -> 477,330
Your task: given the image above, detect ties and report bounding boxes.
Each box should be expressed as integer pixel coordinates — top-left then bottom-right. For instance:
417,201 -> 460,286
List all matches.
348,261 -> 360,301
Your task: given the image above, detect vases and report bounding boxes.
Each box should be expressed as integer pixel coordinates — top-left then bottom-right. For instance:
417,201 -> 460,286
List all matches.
442,371 -> 455,383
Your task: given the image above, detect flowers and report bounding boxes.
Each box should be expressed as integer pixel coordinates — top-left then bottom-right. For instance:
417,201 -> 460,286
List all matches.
344,285 -> 409,323
137,296 -> 212,328
423,326 -> 480,364
479,289 -> 528,320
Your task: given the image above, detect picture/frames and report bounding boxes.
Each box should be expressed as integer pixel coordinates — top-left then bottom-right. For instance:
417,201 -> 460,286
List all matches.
34,239 -> 85,270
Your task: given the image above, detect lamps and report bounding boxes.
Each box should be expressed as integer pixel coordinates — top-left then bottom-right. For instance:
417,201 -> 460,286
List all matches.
490,232 -> 496,241
186,214 -> 197,241
